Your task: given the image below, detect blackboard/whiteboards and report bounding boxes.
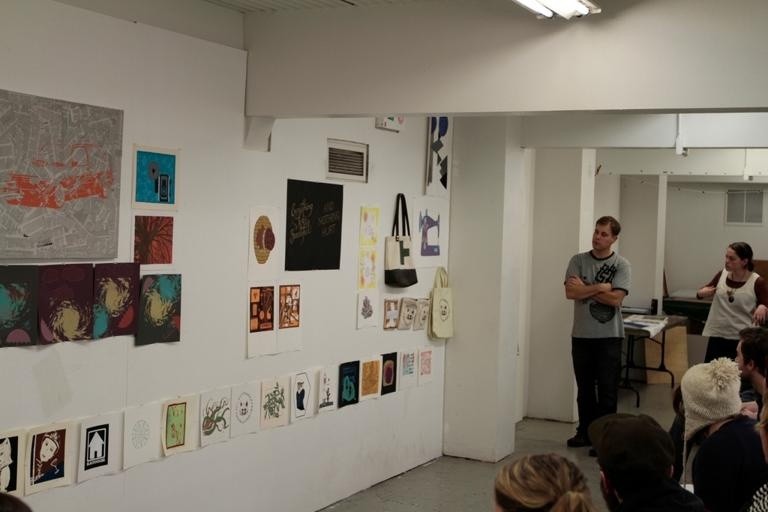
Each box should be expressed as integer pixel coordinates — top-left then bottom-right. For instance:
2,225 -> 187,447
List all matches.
285,178 -> 343,271
1,88 -> 124,261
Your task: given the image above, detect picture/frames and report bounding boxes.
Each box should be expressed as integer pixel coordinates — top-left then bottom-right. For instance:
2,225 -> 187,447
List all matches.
130,143 -> 180,211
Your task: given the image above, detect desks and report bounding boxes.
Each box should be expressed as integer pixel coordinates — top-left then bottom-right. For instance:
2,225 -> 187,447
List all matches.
617,314 -> 690,408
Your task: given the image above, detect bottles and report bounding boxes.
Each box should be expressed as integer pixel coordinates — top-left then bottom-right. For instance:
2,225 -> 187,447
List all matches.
651,297 -> 658,315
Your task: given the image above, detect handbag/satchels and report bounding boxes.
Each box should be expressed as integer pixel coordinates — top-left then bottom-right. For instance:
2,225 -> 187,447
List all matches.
427,265 -> 456,341
384,192 -> 418,289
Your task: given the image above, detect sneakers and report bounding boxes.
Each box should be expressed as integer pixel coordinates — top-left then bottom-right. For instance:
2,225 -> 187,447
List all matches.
566,435 -> 598,458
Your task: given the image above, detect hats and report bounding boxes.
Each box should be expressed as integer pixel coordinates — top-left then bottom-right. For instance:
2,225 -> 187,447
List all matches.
589,411 -> 677,496
678,355 -> 746,442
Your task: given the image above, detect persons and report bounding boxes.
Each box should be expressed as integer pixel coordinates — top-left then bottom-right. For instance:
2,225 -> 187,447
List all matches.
563,216 -> 632,458
695,241 -> 768,364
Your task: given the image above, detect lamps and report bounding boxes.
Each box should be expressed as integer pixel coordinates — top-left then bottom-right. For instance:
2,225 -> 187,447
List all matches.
510,0 -> 604,21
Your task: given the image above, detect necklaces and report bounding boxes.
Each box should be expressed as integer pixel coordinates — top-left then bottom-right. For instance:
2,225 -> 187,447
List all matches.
730,270 -> 746,297
